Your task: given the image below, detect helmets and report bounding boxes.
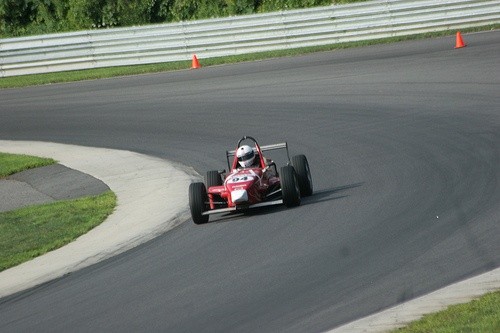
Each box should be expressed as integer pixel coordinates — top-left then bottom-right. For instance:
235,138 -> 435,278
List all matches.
236,144 -> 256,168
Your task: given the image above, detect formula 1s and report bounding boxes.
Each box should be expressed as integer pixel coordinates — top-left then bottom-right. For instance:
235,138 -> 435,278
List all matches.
188,135 -> 313,225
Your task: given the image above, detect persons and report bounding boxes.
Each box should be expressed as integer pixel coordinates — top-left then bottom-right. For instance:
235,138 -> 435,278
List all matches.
236,145 -> 262,169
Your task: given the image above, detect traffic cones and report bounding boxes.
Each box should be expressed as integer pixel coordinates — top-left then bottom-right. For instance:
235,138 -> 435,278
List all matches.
453,31 -> 467,49
190,54 -> 201,69
